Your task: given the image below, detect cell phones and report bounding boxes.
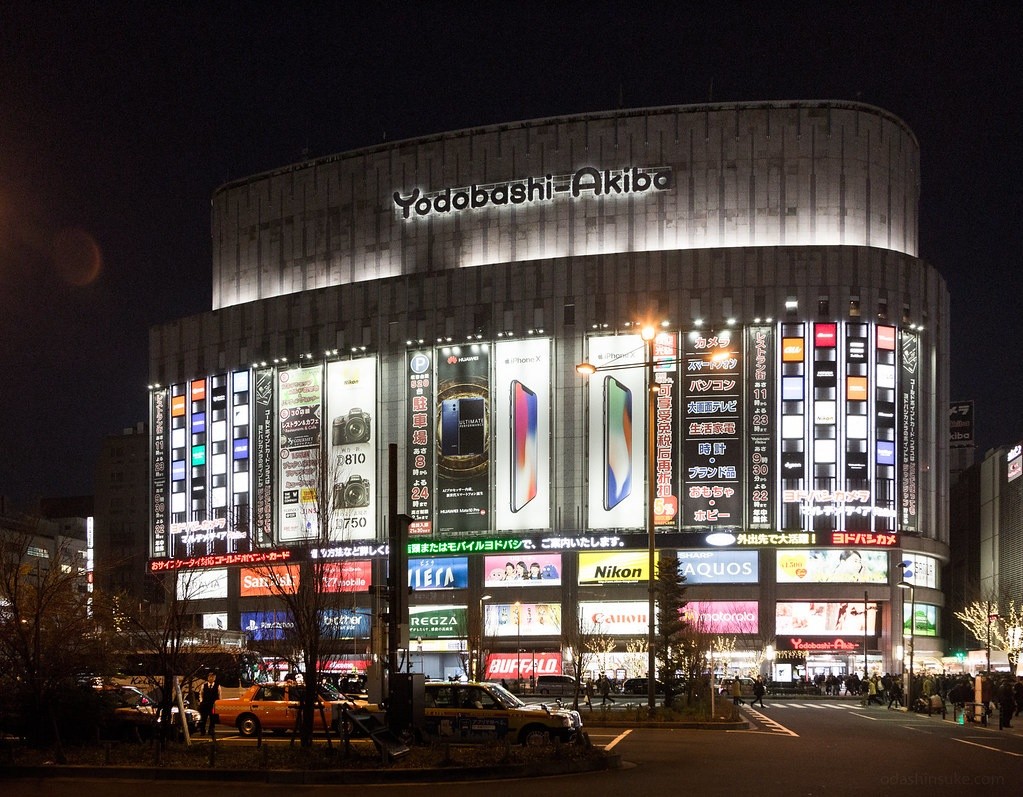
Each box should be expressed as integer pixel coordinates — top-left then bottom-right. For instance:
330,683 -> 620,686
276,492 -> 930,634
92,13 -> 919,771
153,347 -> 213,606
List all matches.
442,400 -> 460,456
457,399 -> 485,455
510,381 -> 538,514
602,375 -> 633,510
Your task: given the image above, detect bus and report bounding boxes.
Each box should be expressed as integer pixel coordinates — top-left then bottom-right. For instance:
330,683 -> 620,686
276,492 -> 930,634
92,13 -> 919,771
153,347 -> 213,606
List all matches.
315,668 -> 427,701
76,647 -> 268,711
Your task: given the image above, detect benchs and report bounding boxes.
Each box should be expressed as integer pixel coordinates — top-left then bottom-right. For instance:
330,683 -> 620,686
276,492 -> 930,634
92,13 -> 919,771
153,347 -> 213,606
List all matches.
916,694 -> 994,726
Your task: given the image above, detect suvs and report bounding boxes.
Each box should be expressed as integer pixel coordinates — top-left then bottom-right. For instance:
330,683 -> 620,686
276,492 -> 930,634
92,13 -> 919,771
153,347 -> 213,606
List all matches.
423,678 -> 583,747
536,675 -> 586,693
621,678 -> 665,696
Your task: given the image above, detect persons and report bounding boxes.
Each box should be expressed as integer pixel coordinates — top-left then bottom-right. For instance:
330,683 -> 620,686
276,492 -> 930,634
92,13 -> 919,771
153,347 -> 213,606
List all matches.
199,673 -> 221,736
448,675 -> 459,681
828,551 -> 862,582
519,674 -> 533,687
501,679 -> 508,690
751,675 -> 766,708
733,676 -> 745,706
585,674 -> 624,705
797,672 -> 1023,730
762,672 -> 773,686
503,562 -> 540,580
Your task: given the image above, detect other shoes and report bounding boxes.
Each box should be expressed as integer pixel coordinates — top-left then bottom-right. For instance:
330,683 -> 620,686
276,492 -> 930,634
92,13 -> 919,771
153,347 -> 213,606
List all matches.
750,701 -> 753,707
614,700 -> 615,704
742,701 -> 745,705
761,705 -> 766,708
600,703 -> 606,705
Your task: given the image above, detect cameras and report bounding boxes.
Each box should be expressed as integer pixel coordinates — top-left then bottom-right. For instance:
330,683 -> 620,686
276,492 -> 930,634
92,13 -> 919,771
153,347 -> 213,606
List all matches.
333,475 -> 371,510
332,408 -> 371,446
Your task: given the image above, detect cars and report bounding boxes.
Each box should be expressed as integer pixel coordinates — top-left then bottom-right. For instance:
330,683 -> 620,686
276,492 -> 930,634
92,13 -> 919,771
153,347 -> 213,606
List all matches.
209,680 -> 383,738
87,686 -> 199,740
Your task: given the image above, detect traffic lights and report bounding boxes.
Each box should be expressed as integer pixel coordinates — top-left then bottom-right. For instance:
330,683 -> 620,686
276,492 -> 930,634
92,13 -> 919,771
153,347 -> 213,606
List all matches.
955,651 -> 968,657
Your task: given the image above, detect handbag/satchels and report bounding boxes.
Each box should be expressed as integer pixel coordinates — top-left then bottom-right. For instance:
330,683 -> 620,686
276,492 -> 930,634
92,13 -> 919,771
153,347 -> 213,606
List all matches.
583,694 -> 589,702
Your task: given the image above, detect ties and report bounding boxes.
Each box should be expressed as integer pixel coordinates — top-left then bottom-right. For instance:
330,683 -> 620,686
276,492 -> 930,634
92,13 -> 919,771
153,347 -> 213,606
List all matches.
210,684 -> 212,688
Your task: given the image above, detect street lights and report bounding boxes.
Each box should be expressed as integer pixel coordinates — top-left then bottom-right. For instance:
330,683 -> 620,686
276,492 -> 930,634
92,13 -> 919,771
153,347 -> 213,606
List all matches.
514,602 -> 521,694
479,593 -> 492,702
896,582 -> 915,709
896,563 -> 906,689
575,327 -> 731,719
850,607 -> 879,677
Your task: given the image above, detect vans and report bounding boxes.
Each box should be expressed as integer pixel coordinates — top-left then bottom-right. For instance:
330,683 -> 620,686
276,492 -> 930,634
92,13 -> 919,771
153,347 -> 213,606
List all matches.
718,677 -> 767,697
